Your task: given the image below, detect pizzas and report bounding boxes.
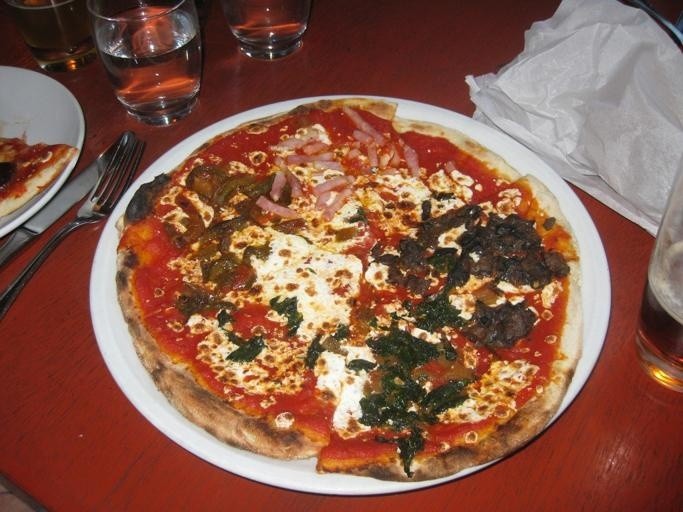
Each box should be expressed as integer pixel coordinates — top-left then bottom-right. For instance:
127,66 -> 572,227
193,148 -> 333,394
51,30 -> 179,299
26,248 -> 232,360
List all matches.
0,138 -> 78,217
116,98 -> 583,481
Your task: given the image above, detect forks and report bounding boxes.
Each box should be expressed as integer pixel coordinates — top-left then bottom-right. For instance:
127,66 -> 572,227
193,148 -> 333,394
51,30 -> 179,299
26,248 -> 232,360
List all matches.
1,135 -> 145,330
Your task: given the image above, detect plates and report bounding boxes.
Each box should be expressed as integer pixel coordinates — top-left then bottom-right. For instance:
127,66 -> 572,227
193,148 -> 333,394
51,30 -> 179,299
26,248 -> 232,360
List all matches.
87,94 -> 613,497
2,64 -> 86,236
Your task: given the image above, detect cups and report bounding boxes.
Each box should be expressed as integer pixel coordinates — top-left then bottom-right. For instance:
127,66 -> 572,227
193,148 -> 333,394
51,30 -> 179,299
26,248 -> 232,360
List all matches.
5,1 -> 101,83
84,1 -> 205,127
635,162 -> 683,393
217,2 -> 310,60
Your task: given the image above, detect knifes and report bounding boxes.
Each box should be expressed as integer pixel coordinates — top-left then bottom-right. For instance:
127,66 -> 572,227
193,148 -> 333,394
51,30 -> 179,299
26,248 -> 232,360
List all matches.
2,132 -> 134,265
